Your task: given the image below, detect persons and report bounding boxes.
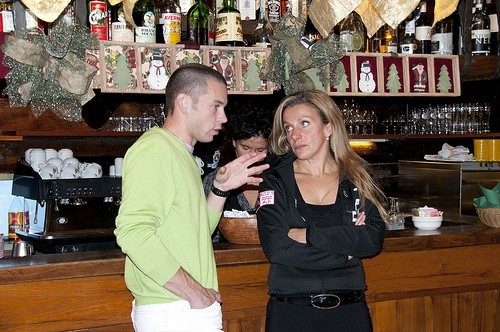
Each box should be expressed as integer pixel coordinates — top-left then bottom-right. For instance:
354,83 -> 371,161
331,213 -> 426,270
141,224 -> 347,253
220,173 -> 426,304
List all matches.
113,64 -> 275,332
256,91 -> 389,331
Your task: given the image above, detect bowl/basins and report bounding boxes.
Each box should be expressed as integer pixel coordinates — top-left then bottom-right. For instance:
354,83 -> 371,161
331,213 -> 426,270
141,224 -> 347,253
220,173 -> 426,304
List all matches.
218,217 -> 261,245
412,216 -> 443,230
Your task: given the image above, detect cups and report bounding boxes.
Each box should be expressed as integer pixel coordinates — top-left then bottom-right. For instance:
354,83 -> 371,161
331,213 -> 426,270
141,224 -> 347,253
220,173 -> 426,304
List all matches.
108,117 -> 152,131
25,148 -> 102,179
407,103 -> 491,134
473,140 -> 500,162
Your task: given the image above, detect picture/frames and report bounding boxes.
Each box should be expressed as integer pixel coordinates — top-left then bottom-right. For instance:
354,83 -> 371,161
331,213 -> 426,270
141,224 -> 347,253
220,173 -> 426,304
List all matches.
99,39 -> 461,98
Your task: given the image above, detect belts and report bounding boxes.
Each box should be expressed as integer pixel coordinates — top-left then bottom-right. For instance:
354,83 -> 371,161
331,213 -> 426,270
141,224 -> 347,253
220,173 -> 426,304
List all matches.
269,291 -> 365,310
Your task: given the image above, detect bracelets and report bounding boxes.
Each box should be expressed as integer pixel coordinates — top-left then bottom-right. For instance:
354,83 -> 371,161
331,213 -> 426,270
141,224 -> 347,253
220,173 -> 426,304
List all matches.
210,184 -> 229,197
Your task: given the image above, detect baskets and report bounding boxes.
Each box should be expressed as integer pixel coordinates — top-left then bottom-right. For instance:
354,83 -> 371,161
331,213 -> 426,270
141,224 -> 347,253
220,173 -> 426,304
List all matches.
475,208 -> 500,228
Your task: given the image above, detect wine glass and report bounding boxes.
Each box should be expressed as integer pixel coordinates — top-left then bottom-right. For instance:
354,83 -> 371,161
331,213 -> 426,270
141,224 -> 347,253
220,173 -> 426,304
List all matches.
336,100 -> 405,135
387,196 -> 399,225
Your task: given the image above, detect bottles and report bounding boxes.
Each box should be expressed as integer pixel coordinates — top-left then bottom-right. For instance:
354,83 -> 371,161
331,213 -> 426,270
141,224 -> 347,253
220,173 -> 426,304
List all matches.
304,0 -> 453,55
249,0 -> 274,47
187,0 -> 215,46
89,0 -> 181,45
24,200 -> 30,228
216,0 -> 244,47
8,195 -> 23,238
471,0 -> 496,58
